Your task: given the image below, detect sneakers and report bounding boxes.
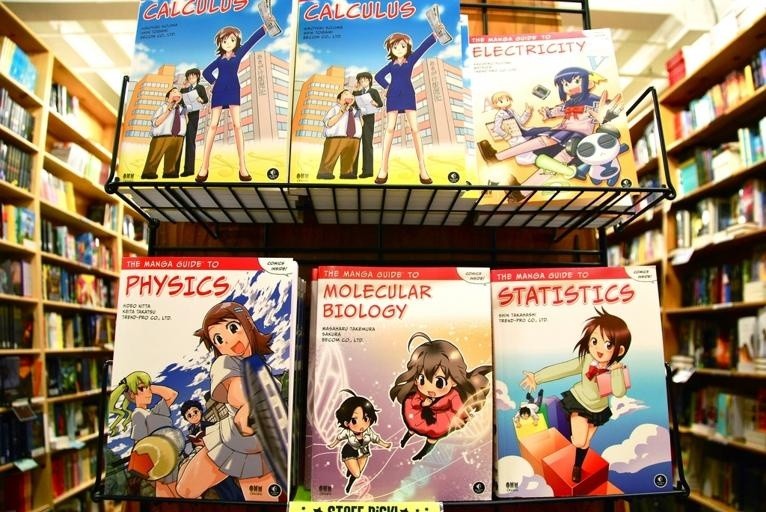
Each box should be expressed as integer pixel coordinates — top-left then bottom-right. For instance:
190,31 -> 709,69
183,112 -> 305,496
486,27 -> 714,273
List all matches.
526,392 -> 530,400
502,176 -> 526,203
538,389 -> 544,396
476,139 -> 502,166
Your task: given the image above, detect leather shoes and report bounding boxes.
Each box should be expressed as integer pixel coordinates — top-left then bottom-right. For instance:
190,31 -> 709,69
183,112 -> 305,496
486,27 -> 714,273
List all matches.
140,171 -> 194,179
194,167 -> 209,181
238,168 -> 252,182
315,171 -> 374,180
374,167 -> 389,184
419,171 -> 433,185
572,463 -> 582,483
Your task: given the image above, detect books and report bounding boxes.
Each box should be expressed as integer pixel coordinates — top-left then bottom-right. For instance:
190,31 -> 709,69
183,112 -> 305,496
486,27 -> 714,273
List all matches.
119,1 -> 639,227
606,2 -> 765,511
106,255 -> 674,501
1,37 -> 142,512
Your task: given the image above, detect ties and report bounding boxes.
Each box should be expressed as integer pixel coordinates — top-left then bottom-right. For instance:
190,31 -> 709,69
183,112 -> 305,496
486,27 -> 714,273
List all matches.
170,106 -> 182,136
188,85 -> 193,91
346,109 -> 356,137
361,88 -> 366,94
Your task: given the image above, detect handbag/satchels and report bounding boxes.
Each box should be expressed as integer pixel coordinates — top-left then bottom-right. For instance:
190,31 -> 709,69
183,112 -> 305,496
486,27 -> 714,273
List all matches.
596,366 -> 632,397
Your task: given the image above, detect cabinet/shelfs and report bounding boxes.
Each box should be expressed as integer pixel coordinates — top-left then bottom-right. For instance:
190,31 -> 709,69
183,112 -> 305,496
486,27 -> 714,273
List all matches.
85,73 -> 695,509
595,13 -> 765,510
0,9 -> 149,510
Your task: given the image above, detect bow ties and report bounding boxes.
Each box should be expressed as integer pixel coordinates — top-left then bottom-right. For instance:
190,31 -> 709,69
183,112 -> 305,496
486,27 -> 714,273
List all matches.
586,363 -> 611,382
